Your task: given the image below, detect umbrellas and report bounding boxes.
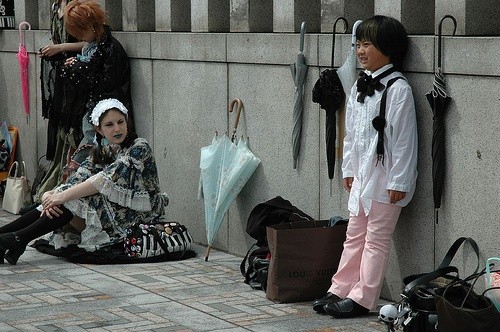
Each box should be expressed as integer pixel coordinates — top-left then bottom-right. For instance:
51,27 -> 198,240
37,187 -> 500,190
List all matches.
425,15 -> 457,224
16,21 -> 32,124
312,17 -> 346,196
290,21 -> 308,169
337,20 -> 363,107
197,98 -> 261,262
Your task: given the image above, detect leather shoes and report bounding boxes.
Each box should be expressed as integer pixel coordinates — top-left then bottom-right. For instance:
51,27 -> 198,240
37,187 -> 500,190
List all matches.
313,292 -> 342,314
323,297 -> 370,319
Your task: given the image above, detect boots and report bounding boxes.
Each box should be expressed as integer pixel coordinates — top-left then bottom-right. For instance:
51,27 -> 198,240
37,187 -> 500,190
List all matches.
0,204 -> 73,265
0,208 -> 45,265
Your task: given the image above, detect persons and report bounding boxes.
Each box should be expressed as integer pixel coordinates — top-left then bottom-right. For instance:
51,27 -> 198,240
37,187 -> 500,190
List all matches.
19,0 -> 138,216
313,15 -> 418,318
0,98 -> 169,265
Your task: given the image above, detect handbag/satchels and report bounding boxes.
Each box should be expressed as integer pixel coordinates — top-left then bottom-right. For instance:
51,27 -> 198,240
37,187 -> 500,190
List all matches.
435,263 -> 500,332
123,221 -> 193,258
30,156 -> 52,205
245,195 -> 316,245
265,218 -> 349,304
2,160 -> 26,215
378,237 -> 480,332
245,249 -> 270,291
